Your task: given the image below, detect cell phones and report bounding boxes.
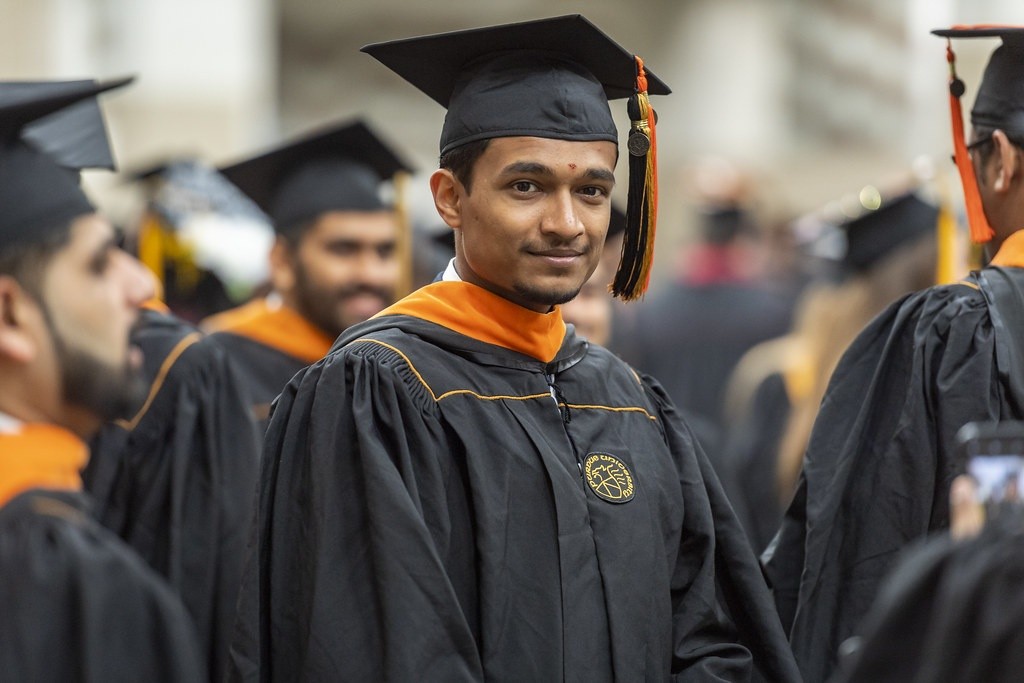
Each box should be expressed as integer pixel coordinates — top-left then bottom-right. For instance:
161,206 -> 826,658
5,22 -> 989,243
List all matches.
955,422 -> 1024,521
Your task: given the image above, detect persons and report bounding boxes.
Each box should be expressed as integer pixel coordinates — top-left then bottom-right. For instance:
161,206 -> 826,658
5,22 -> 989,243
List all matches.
833,468 -> 1024,683
201,116 -> 418,374
728,184 -> 942,496
977,473 -> 1023,533
619,198 -> 787,421
260,13 -> 806,682
117,163 -> 214,310
0,71 -> 207,682
431,189 -> 628,345
774,22 -> 1024,666
78,310 -> 259,679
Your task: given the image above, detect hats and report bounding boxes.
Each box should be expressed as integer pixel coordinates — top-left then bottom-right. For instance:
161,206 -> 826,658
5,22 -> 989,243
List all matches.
358,15 -> 672,303
931,26 -> 1021,248
214,120 -> 412,231
0,78 -> 139,245
